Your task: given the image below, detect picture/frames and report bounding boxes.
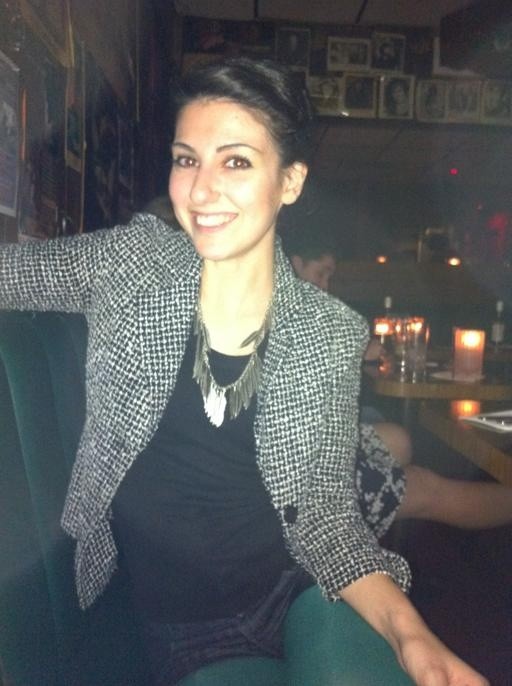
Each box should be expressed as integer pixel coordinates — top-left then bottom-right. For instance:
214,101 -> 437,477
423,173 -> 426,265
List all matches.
370,32 -> 408,72
304,76 -> 340,119
177,13 -> 310,119
340,70 -> 377,121
324,34 -> 371,74
378,74 -> 416,121
1,0 -> 158,246
419,77 -> 511,129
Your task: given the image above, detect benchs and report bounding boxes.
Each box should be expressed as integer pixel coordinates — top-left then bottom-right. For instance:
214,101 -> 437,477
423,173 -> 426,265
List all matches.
0,308 -> 153,686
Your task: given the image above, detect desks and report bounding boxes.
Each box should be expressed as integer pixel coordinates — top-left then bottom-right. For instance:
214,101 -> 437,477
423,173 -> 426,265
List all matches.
366,336 -> 511,554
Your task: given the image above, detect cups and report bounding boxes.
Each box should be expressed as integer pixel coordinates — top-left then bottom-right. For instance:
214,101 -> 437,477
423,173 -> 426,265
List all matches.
391,319 -> 431,372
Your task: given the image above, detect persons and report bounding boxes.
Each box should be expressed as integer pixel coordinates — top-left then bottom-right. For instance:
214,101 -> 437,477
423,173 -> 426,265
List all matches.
280,32 -> 307,64
314,77 -> 336,111
387,77 -> 410,116
282,224 -> 511,542
377,40 -> 397,72
2,48 -> 494,686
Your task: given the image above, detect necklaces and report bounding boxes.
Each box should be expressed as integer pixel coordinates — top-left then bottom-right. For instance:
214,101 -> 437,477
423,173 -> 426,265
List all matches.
191,302 -> 268,428
